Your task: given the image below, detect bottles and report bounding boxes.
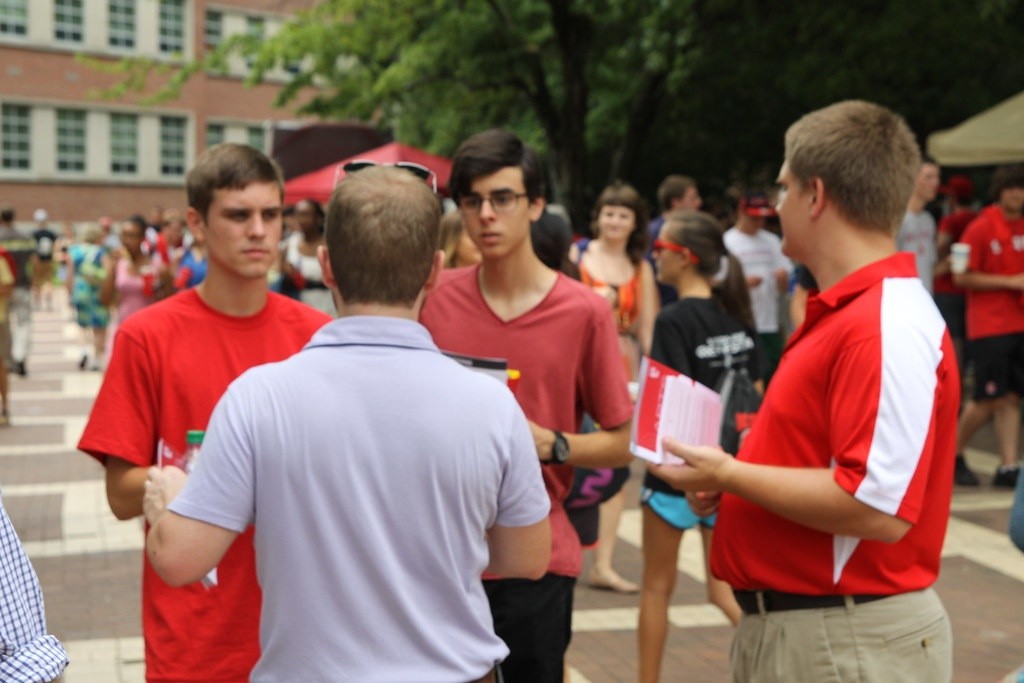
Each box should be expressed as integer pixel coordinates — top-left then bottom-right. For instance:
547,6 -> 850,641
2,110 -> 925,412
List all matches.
175,428 -> 207,480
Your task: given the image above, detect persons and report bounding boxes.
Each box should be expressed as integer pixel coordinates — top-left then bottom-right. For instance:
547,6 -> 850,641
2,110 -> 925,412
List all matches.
0,488 -> 69,683
142,161 -> 552,683
0,207 -> 208,426
895,155 -> 1024,683
436,211 -> 482,267
419,130 -> 638,682
637,212 -> 764,683
77,144 -> 334,683
646,101 -> 961,683
530,174 -> 817,594
267,198 -> 339,319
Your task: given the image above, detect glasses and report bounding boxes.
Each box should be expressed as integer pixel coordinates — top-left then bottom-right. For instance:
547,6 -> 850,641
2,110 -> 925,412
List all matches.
332,159 -> 438,196
651,239 -> 699,265
462,190 -> 530,214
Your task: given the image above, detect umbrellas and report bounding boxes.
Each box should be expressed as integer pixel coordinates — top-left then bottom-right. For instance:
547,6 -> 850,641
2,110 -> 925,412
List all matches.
927,90 -> 1024,165
283,142 -> 454,202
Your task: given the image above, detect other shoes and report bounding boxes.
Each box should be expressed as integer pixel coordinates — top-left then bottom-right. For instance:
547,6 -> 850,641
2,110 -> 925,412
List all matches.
953,452 -> 981,487
990,465 -> 1018,490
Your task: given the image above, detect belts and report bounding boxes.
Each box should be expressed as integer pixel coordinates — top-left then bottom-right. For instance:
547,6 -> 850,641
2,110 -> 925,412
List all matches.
734,589 -> 886,616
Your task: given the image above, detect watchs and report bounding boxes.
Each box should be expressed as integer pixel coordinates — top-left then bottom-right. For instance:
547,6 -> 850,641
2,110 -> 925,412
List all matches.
543,431 -> 570,465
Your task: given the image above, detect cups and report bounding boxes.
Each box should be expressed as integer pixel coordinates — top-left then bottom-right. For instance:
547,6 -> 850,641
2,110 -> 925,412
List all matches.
949,242 -> 972,274
